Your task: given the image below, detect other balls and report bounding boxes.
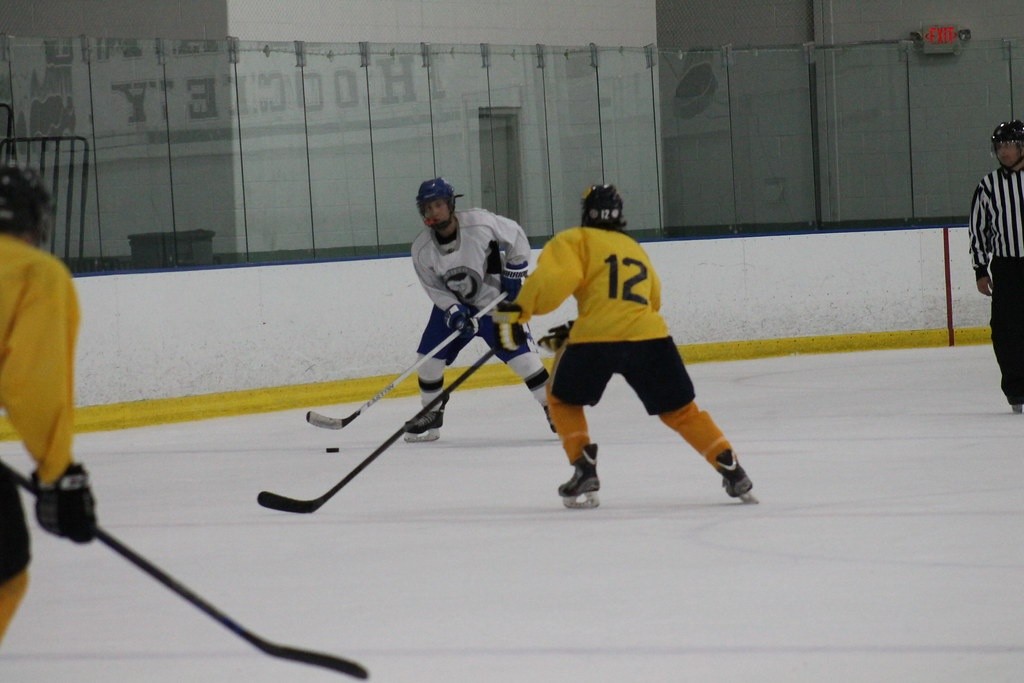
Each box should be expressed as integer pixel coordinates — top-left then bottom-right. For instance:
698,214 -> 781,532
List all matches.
326,447 -> 339,453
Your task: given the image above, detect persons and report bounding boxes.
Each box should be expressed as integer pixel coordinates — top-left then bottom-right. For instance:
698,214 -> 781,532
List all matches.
404,179 -> 558,440
968,121 -> 1024,412
494,184 -> 758,509
0,164 -> 97,641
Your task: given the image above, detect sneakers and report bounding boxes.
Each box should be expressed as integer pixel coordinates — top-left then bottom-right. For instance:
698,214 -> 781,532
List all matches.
716,451 -> 758,504
403,390 -> 449,441
558,444 -> 600,509
542,403 -> 560,437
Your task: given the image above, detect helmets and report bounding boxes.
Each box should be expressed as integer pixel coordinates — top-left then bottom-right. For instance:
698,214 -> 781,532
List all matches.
580,183 -> 623,227
0,164 -> 47,236
415,177 -> 455,229
991,119 -> 1024,168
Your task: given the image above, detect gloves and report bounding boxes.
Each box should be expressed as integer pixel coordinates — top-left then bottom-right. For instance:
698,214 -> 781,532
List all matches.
444,303 -> 480,337
537,321 -> 576,353
493,304 -> 526,351
498,261 -> 530,302
32,463 -> 98,546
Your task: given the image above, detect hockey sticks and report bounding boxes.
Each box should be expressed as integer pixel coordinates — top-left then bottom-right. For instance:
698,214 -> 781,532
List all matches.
256,348 -> 495,515
305,274 -> 526,430
0,459 -> 370,680
